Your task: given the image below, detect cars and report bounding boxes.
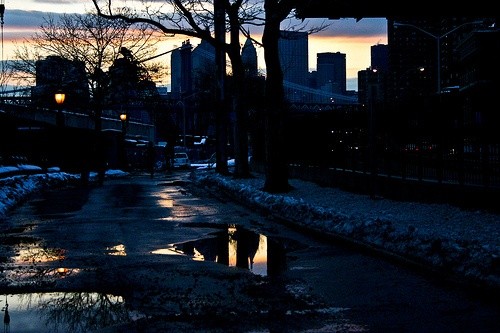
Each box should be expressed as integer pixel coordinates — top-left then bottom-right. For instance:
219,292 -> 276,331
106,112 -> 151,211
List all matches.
172,153 -> 191,169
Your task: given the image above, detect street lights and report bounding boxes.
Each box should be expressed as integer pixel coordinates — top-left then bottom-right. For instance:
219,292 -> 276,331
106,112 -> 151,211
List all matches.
392,20 -> 485,92
175,100 -> 186,147
53,89 -> 66,124
119,110 -> 128,137
